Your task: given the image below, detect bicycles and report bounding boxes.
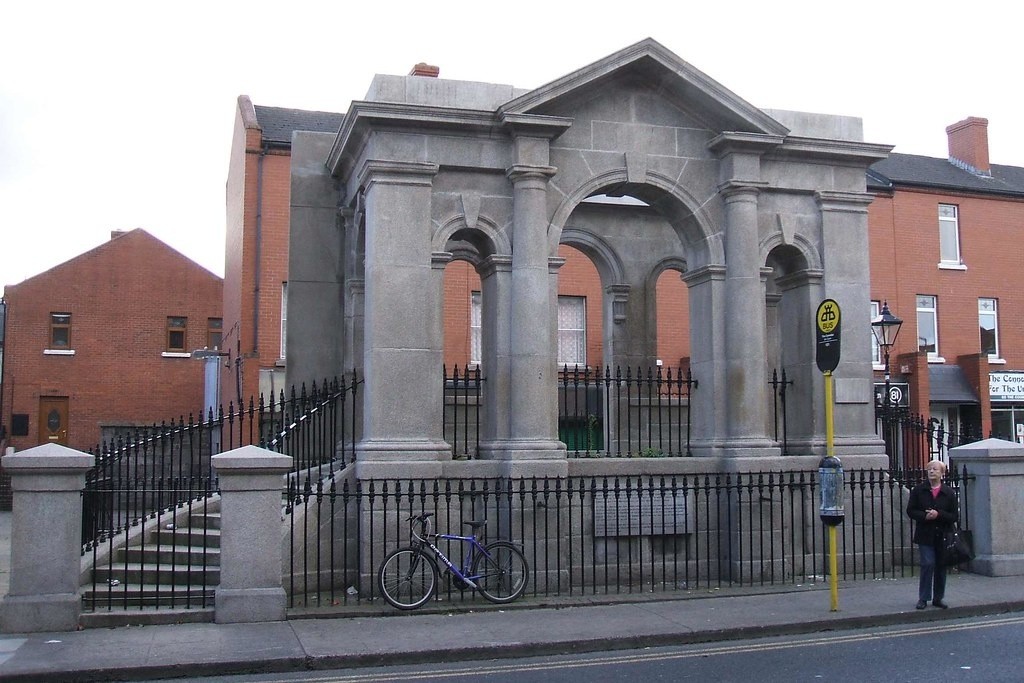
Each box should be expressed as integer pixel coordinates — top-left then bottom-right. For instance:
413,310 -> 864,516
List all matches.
378,513 -> 531,610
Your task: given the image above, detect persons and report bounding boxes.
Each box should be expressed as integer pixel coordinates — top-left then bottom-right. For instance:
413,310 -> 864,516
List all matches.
906,460 -> 958,609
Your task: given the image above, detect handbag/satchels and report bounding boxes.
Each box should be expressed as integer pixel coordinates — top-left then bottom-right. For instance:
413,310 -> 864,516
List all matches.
937,519 -> 976,568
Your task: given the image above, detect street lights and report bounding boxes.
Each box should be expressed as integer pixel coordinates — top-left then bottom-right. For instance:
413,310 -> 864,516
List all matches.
871,299 -> 903,466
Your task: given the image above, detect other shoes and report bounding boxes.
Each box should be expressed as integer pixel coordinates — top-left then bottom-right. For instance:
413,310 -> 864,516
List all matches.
932,599 -> 947,609
916,600 -> 927,609
